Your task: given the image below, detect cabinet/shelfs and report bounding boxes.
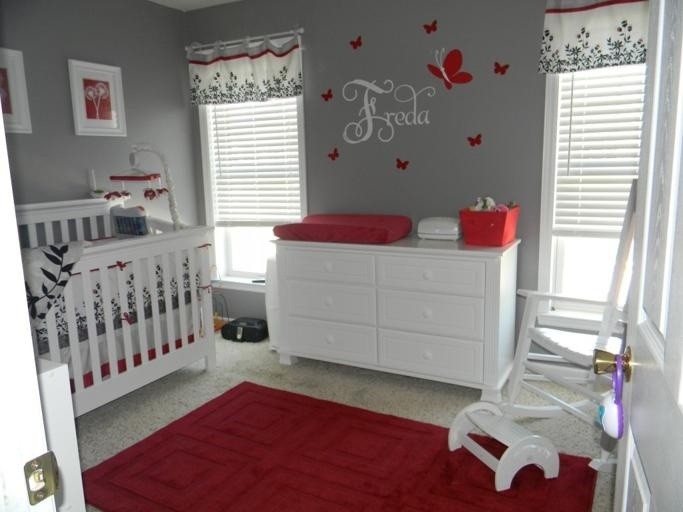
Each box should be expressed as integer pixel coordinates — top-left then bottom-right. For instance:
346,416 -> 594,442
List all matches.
271,238 -> 520,402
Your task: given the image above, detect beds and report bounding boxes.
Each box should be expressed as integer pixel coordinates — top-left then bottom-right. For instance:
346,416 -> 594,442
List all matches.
15,197 -> 216,419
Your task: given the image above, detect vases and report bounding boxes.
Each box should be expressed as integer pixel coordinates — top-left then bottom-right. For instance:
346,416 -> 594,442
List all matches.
459,204 -> 520,248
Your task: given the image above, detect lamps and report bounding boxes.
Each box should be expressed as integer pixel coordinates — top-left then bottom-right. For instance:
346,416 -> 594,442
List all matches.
110,142 -> 181,232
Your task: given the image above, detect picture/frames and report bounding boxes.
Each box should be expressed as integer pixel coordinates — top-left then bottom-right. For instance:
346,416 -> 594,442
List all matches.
1,49 -> 34,135
68,59 -> 127,137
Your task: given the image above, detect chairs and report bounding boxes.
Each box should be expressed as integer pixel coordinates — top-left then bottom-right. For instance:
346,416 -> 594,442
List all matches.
505,179 -> 637,404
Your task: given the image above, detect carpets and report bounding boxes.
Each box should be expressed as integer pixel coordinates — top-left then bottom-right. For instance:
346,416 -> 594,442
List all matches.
82,382 -> 597,511
